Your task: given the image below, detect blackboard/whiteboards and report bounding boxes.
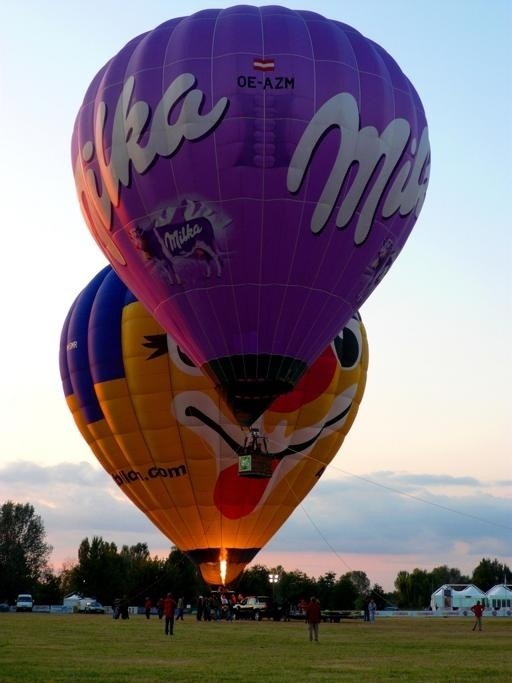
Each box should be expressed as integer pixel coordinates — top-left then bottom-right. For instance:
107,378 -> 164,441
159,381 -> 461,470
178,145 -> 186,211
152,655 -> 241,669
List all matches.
63,591 -> 105,615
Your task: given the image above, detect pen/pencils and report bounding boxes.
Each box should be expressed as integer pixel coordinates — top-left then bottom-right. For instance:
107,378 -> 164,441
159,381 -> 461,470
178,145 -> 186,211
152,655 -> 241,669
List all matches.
16,594 -> 34,611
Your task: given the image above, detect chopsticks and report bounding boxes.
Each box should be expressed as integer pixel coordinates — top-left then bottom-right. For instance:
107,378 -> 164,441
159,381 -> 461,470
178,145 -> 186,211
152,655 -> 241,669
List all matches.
72,5 -> 430,478
59,262 -> 370,608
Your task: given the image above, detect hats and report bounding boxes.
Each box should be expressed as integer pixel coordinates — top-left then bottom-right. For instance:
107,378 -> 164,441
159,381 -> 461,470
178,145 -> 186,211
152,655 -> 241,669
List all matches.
0,604 -> 10,611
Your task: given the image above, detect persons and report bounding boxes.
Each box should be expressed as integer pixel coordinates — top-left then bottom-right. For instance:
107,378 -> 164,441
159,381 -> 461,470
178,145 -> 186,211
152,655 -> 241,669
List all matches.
368,598 -> 377,624
361,598 -> 369,621
305,597 -> 321,641
163,592 -> 178,636
110,591 -> 308,626
470,600 -> 484,631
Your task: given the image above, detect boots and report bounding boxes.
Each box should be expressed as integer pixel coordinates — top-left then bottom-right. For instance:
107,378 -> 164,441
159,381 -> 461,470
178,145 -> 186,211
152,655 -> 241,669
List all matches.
232,596 -> 275,620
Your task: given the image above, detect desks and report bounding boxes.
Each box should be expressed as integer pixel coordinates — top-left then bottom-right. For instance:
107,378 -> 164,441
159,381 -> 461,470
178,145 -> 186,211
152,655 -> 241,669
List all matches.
267,566 -> 279,597
